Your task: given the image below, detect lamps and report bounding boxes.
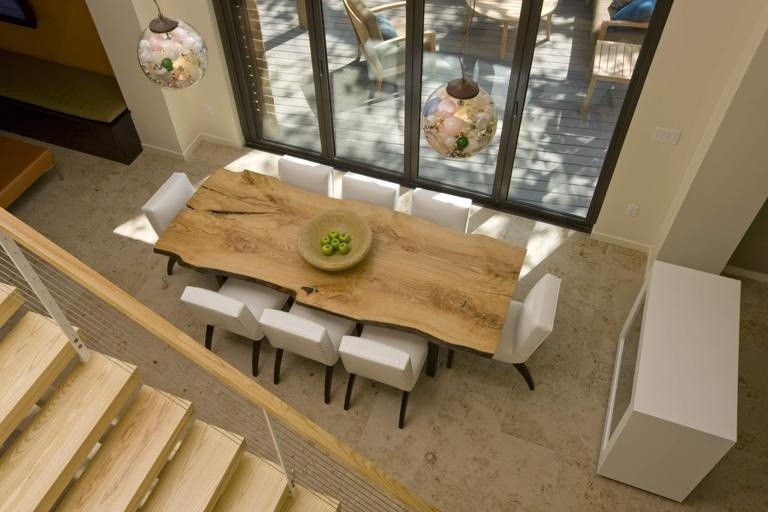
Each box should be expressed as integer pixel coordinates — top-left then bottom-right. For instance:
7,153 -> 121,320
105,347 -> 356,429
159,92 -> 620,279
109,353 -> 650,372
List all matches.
420,1 -> 500,159
135,0 -> 207,92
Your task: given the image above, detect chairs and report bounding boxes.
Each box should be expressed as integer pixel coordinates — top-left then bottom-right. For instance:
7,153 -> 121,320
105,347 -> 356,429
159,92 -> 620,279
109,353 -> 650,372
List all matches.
580,19 -> 649,111
343,1 -> 437,98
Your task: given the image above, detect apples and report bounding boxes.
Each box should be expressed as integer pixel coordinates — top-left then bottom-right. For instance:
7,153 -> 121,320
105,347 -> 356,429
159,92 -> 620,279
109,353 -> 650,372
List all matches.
320,231 -> 350,256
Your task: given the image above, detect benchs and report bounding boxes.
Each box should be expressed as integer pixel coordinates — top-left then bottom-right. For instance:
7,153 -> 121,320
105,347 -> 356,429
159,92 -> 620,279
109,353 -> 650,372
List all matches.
1,51 -> 142,165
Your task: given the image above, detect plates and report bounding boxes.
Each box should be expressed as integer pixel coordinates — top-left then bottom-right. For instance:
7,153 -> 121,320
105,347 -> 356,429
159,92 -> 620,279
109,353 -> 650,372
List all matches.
295,207 -> 376,273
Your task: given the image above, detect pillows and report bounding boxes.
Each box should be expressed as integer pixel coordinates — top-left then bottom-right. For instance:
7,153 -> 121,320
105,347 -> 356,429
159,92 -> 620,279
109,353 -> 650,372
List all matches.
376,15 -> 401,47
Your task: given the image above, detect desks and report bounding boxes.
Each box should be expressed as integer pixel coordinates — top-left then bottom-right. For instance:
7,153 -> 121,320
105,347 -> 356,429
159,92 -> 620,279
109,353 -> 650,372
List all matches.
458,1 -> 559,60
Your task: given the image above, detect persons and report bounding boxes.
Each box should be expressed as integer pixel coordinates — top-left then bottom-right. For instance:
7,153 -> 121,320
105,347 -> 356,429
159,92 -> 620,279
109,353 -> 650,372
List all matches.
362,39 -> 404,132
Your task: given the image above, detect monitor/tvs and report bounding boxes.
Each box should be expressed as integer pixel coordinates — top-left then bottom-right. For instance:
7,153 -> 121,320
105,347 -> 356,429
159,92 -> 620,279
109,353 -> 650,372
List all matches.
0,0 -> 37,29
